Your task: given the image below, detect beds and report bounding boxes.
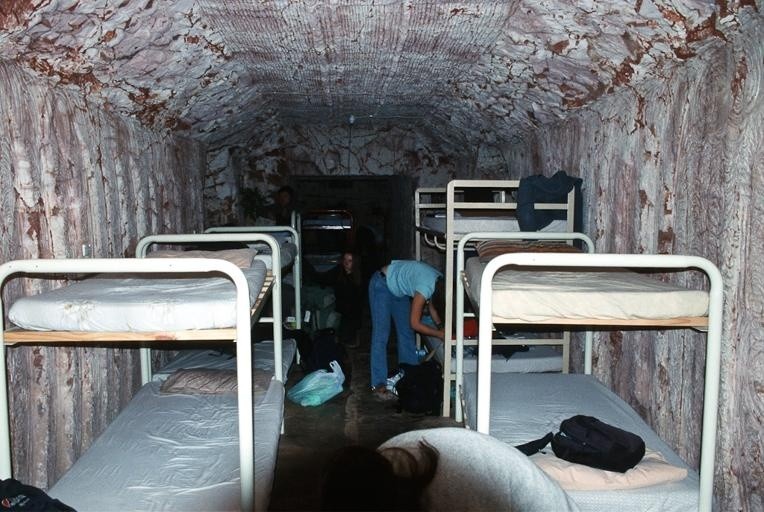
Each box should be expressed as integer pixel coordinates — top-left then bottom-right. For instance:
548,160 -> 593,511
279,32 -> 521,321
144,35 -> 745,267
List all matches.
292,208 -> 355,340
0,233 -> 284,510
454,232 -> 724,511
133,226 -> 303,435
415,182 -> 577,420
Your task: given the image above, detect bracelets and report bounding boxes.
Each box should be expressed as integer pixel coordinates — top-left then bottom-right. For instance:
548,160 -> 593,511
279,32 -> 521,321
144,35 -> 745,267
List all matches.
433,320 -> 444,330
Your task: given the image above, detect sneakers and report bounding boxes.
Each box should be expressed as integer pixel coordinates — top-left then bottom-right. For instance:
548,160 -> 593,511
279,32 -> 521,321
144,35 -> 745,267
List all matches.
371,386 -> 401,402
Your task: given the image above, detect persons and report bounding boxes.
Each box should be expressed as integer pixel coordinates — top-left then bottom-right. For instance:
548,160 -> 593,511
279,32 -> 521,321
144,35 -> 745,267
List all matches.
365,258 -> 446,401
262,425 -> 581,511
275,183 -> 306,215
307,247 -> 373,353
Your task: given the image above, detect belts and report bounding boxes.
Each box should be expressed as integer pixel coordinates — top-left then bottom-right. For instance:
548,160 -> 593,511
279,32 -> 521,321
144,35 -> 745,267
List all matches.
379,270 -> 386,279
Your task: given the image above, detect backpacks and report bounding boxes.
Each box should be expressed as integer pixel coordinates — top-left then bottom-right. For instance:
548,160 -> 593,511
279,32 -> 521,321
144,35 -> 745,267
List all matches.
301,285 -> 339,337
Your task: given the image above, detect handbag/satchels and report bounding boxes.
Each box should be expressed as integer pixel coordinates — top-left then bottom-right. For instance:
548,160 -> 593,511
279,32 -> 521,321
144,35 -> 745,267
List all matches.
550,412 -> 649,473
397,357 -> 446,415
286,360 -> 345,407
